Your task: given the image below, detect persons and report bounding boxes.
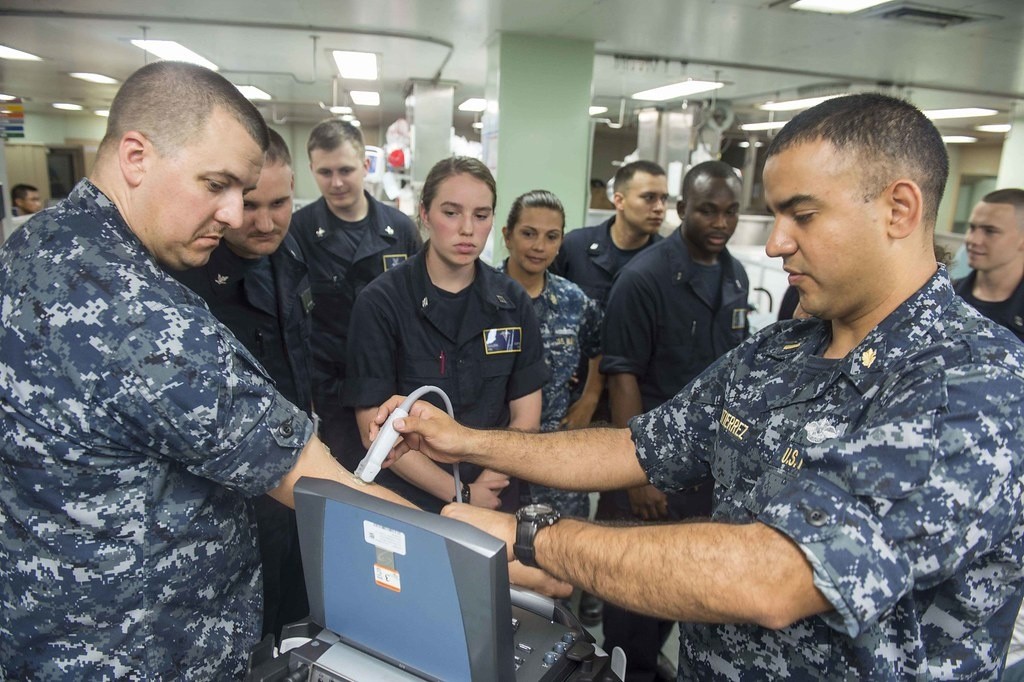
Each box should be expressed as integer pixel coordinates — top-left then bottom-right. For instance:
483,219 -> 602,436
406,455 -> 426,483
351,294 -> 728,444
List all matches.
0,61 -> 572,682
495,190 -> 605,607
601,161 -> 750,682
370,93 -> 1024,682
10,184 -> 41,216
339,156 -> 550,514
551,160 -> 668,626
160,129 -> 315,644
290,120 -> 422,456
952,189 -> 1024,343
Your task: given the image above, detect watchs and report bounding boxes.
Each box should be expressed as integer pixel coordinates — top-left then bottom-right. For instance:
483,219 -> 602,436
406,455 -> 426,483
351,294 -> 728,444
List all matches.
513,504 -> 560,568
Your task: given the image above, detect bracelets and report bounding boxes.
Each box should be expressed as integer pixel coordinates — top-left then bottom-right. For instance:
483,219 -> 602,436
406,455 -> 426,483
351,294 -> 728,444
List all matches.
453,483 -> 471,504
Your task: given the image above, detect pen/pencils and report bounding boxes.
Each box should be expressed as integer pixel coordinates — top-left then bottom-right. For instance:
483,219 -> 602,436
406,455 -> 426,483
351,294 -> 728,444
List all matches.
333,274 -> 337,288
691,321 -> 696,335
255,329 -> 263,351
440,351 -> 445,375
